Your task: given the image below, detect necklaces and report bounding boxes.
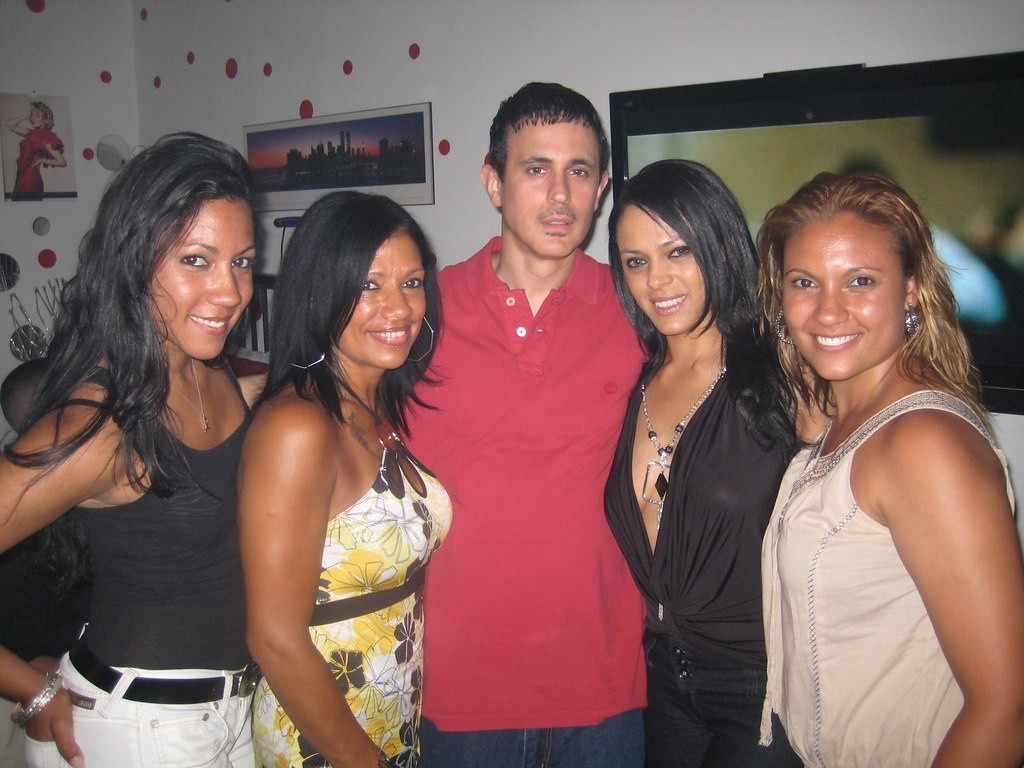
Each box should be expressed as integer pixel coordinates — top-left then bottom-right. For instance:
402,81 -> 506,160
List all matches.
167,358 -> 210,433
641,368 -> 727,528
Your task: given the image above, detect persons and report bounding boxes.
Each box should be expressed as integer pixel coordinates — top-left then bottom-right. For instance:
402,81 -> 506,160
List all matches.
4,100 -> 67,201
603,158 -> 834,768
754,171 -> 1024,768
398,81 -> 652,768
0,132 -> 266,768
238,191 -> 454,768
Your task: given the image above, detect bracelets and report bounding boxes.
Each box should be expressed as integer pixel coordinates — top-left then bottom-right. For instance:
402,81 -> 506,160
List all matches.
376,747 -> 390,768
11,671 -> 63,726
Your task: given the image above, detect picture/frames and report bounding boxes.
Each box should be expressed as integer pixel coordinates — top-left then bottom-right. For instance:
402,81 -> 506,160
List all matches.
610,48 -> 1024,415
244,102 -> 437,212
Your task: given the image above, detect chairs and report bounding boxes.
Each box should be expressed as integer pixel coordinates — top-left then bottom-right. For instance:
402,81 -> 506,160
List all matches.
229,272 -> 279,353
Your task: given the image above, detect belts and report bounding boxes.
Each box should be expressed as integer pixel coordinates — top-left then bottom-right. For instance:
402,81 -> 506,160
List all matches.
69,633 -> 264,704
